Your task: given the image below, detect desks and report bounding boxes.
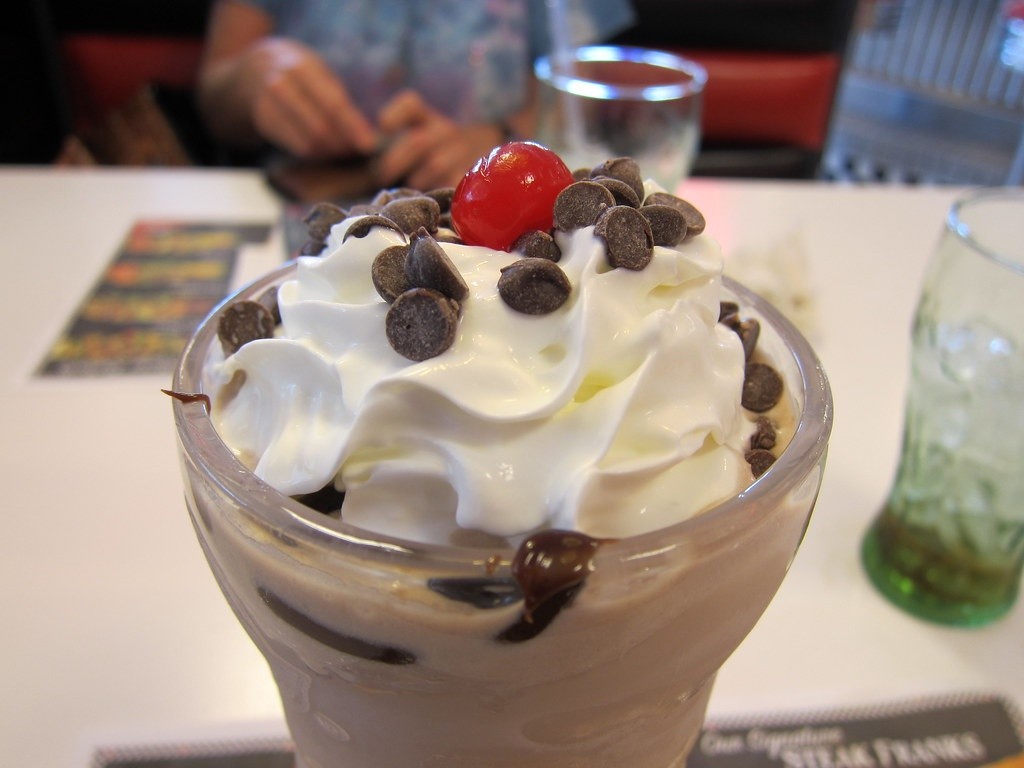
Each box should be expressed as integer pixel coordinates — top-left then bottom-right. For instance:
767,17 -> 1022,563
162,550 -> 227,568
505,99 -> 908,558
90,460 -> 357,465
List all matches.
0,168 -> 1024,768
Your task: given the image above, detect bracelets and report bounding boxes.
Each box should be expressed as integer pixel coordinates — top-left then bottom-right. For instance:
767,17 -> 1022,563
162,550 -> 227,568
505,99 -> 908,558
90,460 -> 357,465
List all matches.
496,119 -> 520,144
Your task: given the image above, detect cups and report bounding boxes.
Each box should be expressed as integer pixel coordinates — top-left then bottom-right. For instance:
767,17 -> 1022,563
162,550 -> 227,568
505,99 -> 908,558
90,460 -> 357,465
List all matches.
859,187 -> 1024,631
529,48 -> 706,191
171,246 -> 828,768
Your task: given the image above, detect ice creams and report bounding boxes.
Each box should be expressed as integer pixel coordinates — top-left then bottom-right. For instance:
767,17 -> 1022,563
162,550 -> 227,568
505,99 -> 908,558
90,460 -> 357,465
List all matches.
162,159 -> 832,768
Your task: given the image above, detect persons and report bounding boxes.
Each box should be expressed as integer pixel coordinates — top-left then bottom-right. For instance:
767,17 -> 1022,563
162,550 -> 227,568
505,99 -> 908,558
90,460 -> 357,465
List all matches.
198,0 -> 637,193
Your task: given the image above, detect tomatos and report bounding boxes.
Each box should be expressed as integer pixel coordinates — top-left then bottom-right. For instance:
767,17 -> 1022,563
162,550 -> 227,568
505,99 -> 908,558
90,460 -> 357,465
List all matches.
449,141 -> 577,253
